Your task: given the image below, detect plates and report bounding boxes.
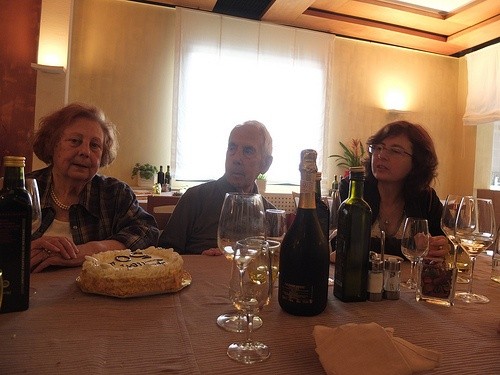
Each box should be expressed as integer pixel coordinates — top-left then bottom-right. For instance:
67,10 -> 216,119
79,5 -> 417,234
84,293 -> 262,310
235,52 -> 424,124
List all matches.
75,268 -> 192,299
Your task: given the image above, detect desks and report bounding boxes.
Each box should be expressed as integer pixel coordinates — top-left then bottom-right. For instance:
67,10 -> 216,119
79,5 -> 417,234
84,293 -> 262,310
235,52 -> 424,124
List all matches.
0,252 -> 500,375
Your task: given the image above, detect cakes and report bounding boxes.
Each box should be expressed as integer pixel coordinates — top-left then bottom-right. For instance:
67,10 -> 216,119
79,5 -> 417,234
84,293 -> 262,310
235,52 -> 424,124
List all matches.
79,246 -> 184,295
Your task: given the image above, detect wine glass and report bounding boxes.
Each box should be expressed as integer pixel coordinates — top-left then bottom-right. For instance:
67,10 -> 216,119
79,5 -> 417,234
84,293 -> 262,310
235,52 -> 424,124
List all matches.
400,218 -> 429,290
455,196 -> 496,304
440,194 -> 477,268
216,192 -> 266,333
225,239 -> 271,365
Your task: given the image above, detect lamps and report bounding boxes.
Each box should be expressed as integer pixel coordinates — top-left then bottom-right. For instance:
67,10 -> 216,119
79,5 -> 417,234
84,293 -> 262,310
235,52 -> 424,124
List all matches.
31,62 -> 65,74
389,110 -> 412,114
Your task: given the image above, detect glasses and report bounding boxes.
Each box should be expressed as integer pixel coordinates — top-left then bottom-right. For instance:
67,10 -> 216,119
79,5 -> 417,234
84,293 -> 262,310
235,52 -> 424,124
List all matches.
368,143 -> 413,159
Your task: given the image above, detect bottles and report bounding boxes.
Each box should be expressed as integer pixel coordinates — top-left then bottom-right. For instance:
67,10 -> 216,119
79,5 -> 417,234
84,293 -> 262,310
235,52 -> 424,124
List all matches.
278,149 -> 330,317
0,155 -> 31,312
153,164 -> 171,194
384,259 -> 400,300
491,226 -> 500,283
332,165 -> 372,303
332,175 -> 339,190
367,260 -> 384,302
316,171 -> 330,243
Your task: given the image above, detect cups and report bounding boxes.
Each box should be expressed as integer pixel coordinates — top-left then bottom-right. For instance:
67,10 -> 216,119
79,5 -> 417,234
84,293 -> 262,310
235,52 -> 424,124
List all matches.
445,241 -> 470,283
265,208 -> 286,243
248,240 -> 280,305
415,260 -> 457,308
24,178 -> 41,234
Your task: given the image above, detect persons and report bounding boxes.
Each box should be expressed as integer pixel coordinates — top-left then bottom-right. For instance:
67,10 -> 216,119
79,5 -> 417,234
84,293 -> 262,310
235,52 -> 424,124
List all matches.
157,120 -> 278,255
24,103 -> 160,272
340,121 -> 462,260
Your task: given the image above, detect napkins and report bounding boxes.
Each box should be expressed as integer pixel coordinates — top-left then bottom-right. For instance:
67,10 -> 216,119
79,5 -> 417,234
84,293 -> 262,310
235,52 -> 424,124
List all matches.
314,322 -> 441,375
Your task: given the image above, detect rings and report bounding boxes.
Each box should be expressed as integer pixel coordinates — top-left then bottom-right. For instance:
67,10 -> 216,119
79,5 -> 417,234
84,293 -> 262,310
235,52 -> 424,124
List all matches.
45,249 -> 51,257
440,246 -> 442,249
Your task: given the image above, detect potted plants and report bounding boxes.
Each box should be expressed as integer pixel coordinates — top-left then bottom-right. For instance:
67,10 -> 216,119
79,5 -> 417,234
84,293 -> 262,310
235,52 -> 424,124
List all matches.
254,174 -> 266,194
131,162 -> 158,187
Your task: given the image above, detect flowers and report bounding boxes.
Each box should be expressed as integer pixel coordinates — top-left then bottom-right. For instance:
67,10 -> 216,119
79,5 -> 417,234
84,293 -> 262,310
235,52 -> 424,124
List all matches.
328,137 -> 370,169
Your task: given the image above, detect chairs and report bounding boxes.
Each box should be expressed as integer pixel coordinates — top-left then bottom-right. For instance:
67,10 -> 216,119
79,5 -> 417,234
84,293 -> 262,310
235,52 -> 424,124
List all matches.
147,196 -> 180,230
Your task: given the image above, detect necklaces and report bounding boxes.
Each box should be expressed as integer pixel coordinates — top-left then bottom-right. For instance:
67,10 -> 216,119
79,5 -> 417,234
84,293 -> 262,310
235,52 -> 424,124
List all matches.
51,191 -> 71,210
385,220 -> 388,223
